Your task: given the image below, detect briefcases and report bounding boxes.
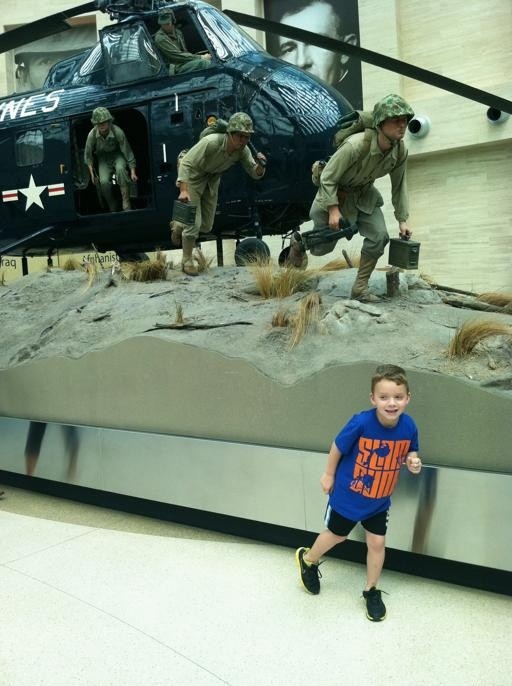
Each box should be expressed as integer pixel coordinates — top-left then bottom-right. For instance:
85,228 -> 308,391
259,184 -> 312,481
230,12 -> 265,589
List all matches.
172,200 -> 198,226
388,234 -> 421,271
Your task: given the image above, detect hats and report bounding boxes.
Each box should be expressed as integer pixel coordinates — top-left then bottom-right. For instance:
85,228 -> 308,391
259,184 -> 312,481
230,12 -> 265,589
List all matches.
158,9 -> 177,25
226,112 -> 255,133
372,94 -> 414,125
90,106 -> 113,124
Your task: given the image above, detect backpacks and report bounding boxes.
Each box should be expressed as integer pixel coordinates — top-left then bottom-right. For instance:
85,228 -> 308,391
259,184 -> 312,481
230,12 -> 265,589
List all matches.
332,108 -> 375,165
200,118 -> 229,156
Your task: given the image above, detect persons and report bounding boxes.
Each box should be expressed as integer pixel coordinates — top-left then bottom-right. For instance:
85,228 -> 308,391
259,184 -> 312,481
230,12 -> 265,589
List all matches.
16,51 -> 77,91
295,364 -> 421,622
84,107 -> 139,212
291,94 -> 415,305
266,0 -> 361,109
154,8 -> 212,74
170,112 -> 266,276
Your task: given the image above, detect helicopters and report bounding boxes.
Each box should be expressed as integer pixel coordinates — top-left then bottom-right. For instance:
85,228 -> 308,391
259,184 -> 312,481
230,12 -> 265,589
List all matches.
0,0 -> 512,275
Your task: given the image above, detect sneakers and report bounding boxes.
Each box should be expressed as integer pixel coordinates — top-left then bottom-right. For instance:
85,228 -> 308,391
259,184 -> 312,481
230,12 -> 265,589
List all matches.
363,588 -> 386,622
295,547 -> 322,595
170,221 -> 184,244
289,234 -> 305,268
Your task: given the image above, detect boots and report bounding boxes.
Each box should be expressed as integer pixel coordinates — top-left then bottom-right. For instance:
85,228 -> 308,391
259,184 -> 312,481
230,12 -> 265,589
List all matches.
181,234 -> 199,277
121,186 -> 131,211
108,197 -> 118,212
350,251 -> 377,302
